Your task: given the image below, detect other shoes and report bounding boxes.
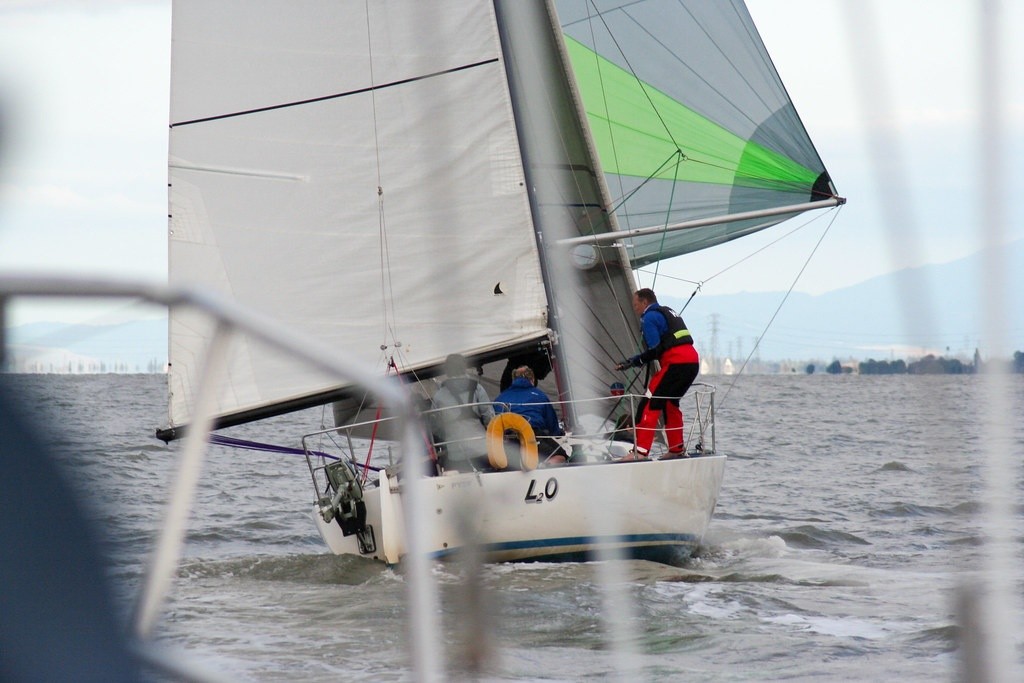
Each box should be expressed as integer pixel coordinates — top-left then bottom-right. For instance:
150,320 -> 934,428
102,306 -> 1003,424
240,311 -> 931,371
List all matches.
619,450 -> 652,462
658,448 -> 689,459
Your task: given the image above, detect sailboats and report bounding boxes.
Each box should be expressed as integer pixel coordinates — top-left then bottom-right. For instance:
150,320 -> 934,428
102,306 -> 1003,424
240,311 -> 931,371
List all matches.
153,1 -> 848,567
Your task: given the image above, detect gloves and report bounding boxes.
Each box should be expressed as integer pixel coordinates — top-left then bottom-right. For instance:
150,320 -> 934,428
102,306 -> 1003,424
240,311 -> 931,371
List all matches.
627,356 -> 643,368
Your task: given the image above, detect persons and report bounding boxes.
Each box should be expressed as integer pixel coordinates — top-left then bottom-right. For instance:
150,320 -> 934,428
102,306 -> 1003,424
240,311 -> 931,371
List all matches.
430,354 -> 495,473
614,287 -> 699,461
497,351 -> 552,390
493,365 -> 571,466
609,415 -> 638,445
